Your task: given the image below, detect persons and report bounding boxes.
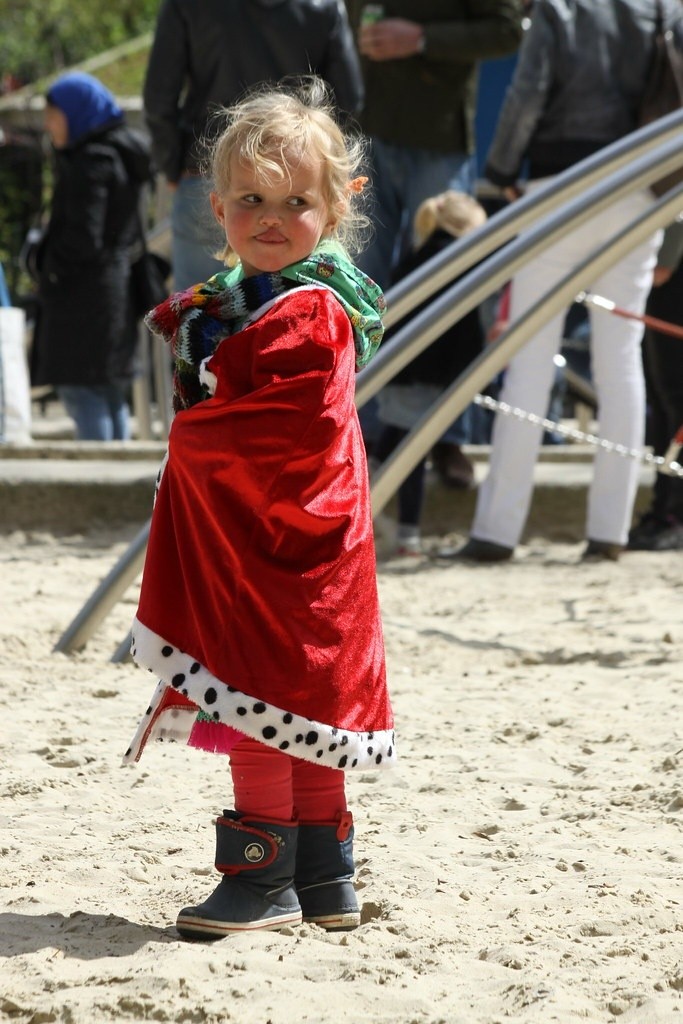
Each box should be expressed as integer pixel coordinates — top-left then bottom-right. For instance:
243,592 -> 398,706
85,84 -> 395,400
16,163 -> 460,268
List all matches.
130,74 -> 394,934
353,0 -> 526,276
25,73 -> 158,439
432,0 -> 683,563
376,191 -> 487,552
142,0 -> 365,291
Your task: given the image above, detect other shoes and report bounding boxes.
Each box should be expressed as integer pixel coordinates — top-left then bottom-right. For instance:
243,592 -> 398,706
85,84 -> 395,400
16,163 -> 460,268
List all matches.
394,527 -> 419,545
581,540 -> 618,561
434,538 -> 512,567
628,512 -> 683,550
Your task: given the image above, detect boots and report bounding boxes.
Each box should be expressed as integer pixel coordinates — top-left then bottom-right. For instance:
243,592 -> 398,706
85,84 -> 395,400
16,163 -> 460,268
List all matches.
294,810 -> 360,928
176,810 -> 302,936
